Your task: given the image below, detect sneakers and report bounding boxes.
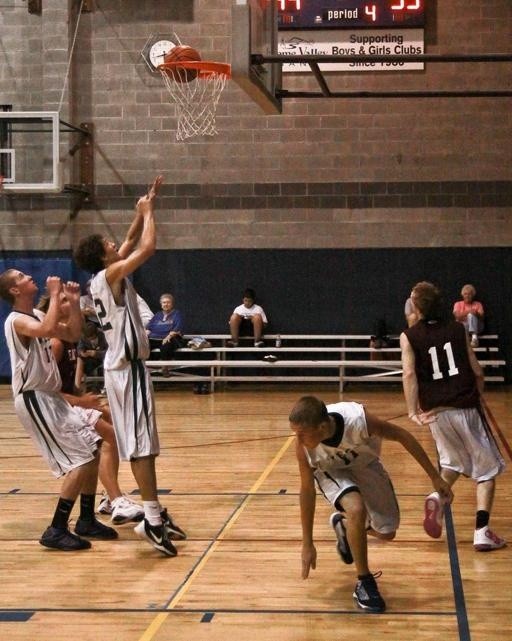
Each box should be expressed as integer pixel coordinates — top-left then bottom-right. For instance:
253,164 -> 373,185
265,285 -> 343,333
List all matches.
109,493 -> 145,526
98,494 -> 112,515
191,382 -> 210,395
470,338 -> 480,349
224,339 -> 241,347
472,524 -> 507,552
133,517 -> 178,557
160,507 -> 187,542
38,526 -> 92,552
422,489 -> 446,540
73,517 -> 119,540
253,340 -> 266,347
161,368 -> 170,378
329,510 -> 355,566
351,569 -> 389,613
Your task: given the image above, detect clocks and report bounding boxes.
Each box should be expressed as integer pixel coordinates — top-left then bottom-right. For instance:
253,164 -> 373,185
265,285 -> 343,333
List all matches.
139,31 -> 184,76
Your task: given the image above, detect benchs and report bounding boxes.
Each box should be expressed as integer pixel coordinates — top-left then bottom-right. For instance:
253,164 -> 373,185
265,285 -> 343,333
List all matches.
128,328 -> 507,398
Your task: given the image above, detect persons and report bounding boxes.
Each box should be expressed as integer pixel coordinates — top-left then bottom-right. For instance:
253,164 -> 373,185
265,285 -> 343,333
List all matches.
453,284 -> 484,347
226,289 -> 268,347
400,282 -> 506,551
288,395 -> 454,614
404,298 -> 422,329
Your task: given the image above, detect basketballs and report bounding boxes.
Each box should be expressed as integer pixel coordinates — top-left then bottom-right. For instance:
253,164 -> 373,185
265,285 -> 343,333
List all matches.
164,45 -> 201,82
